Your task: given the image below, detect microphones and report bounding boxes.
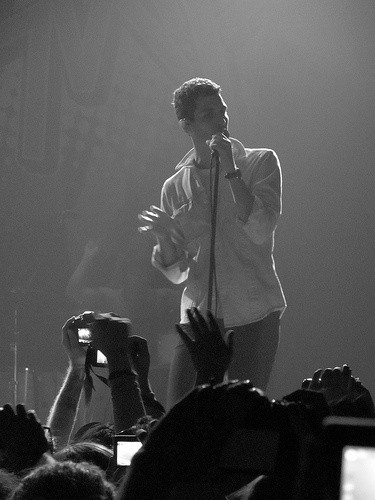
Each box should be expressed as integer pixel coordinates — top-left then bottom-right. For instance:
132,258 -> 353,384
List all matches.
211,130 -> 229,157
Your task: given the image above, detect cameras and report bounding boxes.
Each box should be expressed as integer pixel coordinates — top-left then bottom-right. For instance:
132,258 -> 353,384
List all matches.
302,378 -> 322,390
339,446 -> 375,500
74,311 -> 97,344
92,343 -> 140,367
115,434 -> 143,467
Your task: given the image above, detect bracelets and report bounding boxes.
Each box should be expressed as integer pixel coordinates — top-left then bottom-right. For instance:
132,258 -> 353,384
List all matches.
223,168 -> 241,180
143,392 -> 155,401
109,369 -> 142,400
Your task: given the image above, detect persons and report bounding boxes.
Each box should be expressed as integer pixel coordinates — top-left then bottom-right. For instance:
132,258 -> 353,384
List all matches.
1,304 -> 375,500
137,78 -> 287,418
